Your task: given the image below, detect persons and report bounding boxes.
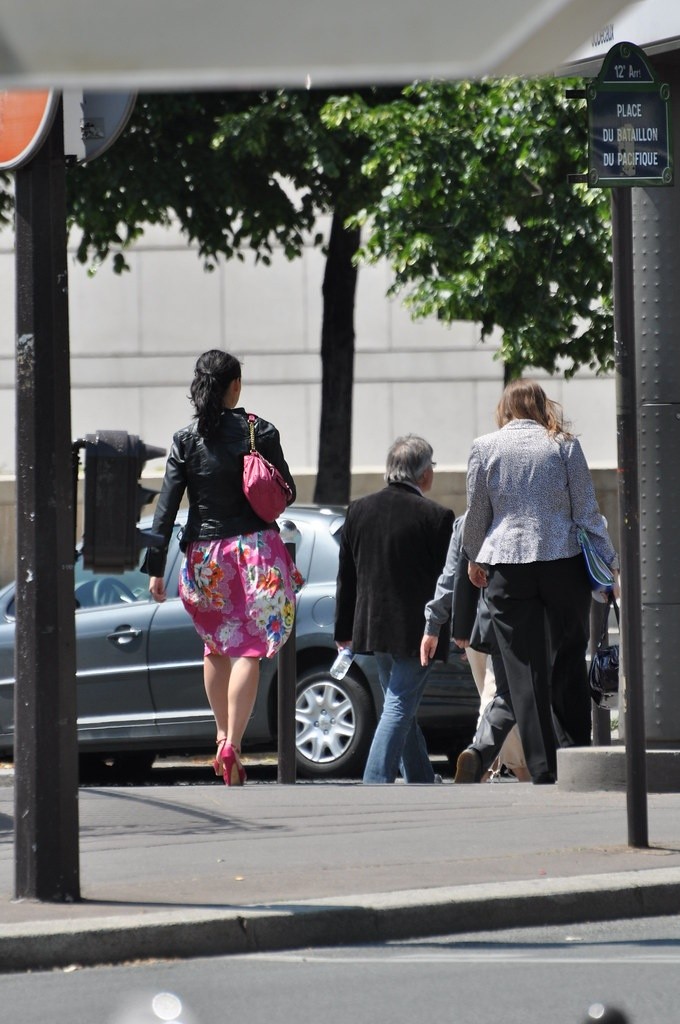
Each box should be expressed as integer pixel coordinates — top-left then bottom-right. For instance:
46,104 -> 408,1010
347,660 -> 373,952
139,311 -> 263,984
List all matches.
461,382 -> 619,784
140,350 -> 305,787
333,435 -> 457,784
420,513 -> 530,783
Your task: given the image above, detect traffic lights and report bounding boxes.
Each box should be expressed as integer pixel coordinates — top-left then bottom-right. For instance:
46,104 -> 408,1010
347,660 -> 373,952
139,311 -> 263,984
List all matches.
81,428 -> 166,575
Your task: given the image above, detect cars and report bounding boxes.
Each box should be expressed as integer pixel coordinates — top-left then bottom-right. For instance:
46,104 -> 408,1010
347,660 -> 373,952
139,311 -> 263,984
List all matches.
0,501 -> 481,784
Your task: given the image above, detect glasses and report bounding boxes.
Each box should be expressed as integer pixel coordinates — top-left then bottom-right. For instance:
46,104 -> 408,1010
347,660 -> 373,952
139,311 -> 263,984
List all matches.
428,461 -> 437,470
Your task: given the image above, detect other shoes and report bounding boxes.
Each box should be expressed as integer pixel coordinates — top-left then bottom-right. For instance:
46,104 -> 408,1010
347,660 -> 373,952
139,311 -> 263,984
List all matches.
455,748 -> 482,784
434,773 -> 443,785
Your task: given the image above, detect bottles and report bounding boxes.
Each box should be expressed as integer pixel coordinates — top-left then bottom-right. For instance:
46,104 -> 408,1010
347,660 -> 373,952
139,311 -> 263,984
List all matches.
329,642 -> 357,681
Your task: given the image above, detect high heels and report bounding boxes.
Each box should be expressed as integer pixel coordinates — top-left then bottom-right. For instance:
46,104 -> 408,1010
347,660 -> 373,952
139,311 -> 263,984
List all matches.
213,738 -> 228,776
221,745 -> 248,787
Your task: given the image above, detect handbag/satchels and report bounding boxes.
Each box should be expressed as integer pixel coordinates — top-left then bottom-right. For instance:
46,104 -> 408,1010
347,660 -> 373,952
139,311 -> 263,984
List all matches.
242,414 -> 293,522
588,645 -> 620,711
578,529 -> 616,586
